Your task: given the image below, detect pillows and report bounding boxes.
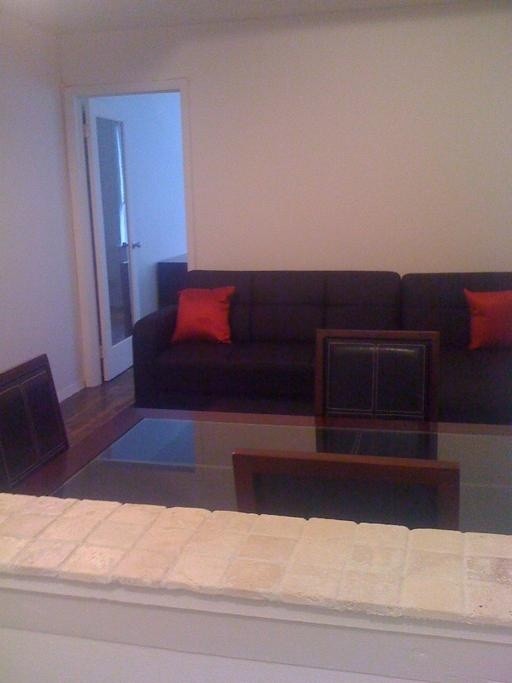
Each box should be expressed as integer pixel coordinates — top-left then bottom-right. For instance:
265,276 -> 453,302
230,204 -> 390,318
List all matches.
460,286 -> 511,349
171,286 -> 236,344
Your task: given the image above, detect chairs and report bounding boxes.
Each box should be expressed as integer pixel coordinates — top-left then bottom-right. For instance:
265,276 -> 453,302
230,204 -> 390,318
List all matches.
316,427 -> 438,461
233,447 -> 460,531
314,326 -> 441,421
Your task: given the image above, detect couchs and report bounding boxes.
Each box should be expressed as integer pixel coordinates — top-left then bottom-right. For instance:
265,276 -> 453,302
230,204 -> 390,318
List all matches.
133,269 -> 400,415
399,273 -> 511,425
